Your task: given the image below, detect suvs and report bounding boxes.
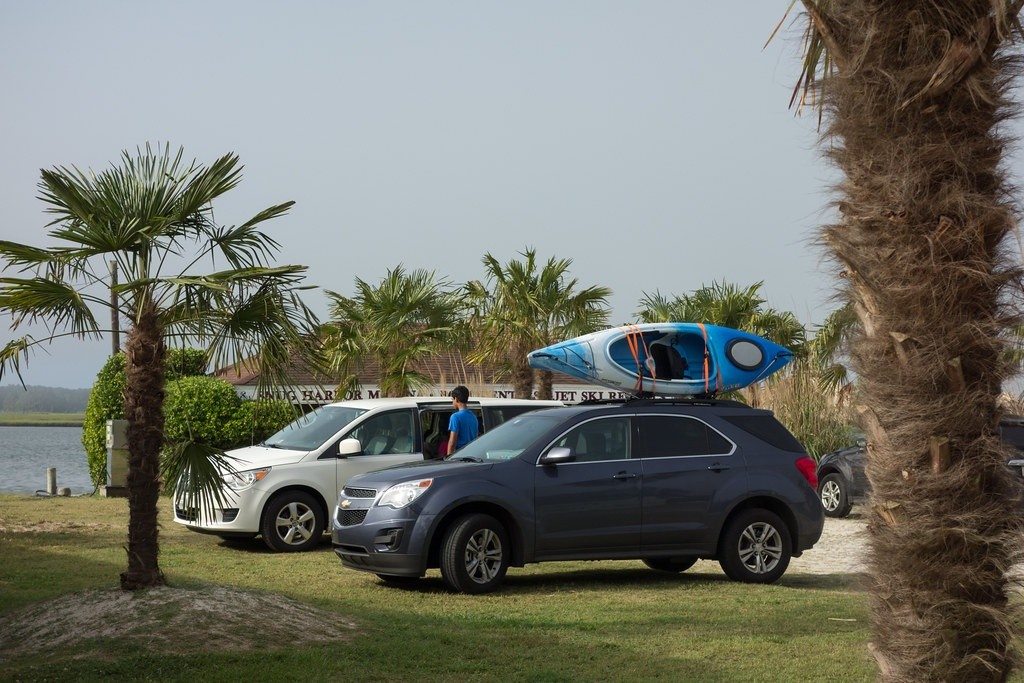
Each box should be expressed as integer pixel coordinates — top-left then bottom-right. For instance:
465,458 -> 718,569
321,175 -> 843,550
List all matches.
327,388 -> 827,594
814,414 -> 1024,519
170,395 -> 589,552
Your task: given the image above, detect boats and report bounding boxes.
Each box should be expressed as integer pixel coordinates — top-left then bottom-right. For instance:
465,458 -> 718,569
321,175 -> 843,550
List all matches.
524,322 -> 796,397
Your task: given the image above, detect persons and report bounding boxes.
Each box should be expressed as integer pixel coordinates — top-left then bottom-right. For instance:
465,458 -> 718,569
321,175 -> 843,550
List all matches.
446,386 -> 479,457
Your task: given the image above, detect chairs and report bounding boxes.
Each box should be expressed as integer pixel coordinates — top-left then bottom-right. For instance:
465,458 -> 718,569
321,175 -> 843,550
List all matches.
364,416 -> 392,454
585,431 -> 607,454
389,414 -> 412,453
651,335 -> 692,380
